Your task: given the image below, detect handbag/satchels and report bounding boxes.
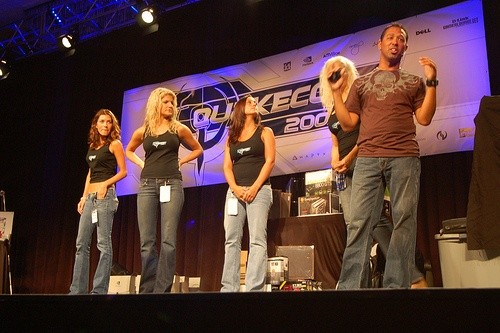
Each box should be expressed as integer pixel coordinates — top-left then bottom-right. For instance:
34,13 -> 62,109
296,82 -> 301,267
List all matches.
0,195 -> 15,242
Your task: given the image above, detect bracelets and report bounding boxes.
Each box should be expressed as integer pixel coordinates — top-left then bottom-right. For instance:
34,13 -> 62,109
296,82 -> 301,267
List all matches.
81,197 -> 86,200
426,80 -> 439,86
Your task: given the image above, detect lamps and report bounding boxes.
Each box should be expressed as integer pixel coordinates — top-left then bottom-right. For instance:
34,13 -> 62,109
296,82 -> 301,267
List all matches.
134,2 -> 164,34
0,45 -> 19,81
56,23 -> 83,57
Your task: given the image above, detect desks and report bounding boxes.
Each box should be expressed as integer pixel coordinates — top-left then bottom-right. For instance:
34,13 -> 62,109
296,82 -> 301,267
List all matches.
246,214 -> 380,287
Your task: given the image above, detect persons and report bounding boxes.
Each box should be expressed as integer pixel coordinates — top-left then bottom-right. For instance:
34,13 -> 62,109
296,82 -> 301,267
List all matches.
327,23 -> 437,289
319,56 -> 427,289
68,109 -> 128,294
221,95 -> 276,293
126,87 -> 203,294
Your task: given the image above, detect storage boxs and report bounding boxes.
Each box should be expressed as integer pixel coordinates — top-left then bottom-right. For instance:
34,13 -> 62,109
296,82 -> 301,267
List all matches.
305,170 -> 338,195
297,193 -> 340,213
